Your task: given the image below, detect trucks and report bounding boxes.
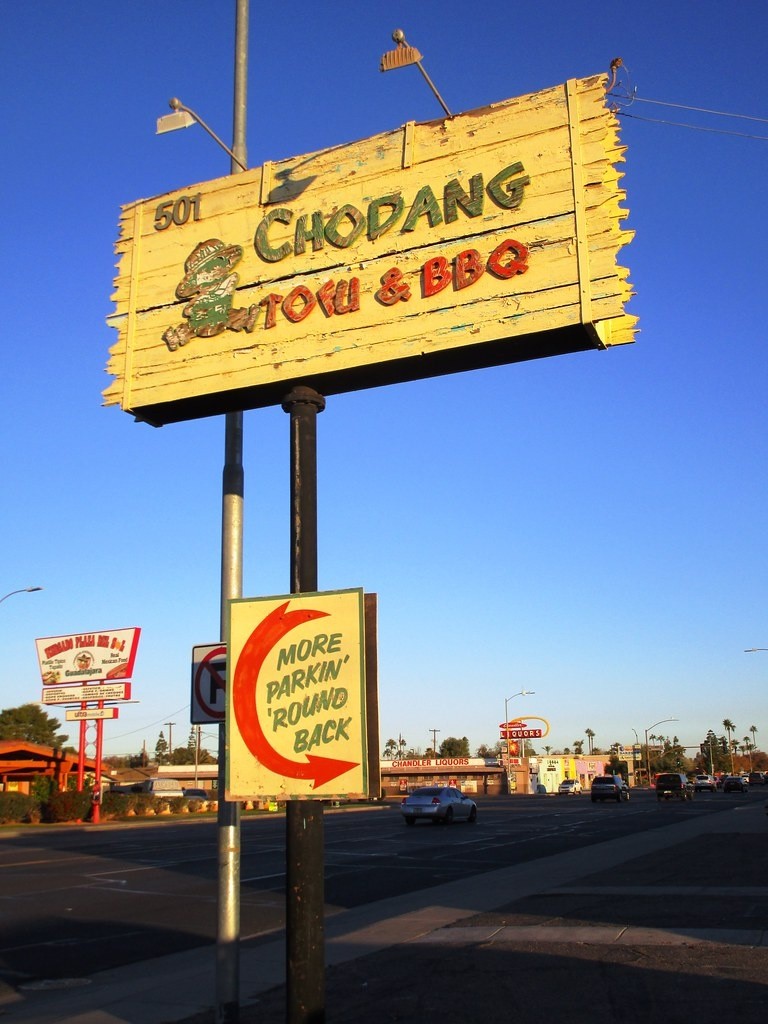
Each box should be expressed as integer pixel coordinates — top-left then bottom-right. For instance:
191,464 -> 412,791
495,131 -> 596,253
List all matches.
109,778 -> 183,798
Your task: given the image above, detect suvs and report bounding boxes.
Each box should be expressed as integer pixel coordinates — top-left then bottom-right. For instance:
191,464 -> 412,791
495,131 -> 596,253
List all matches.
591,775 -> 630,802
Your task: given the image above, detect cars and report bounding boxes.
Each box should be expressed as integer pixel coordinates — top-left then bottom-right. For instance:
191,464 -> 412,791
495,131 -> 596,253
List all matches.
712,777 -> 722,789
400,787 -> 477,825
742,774 -> 749,783
750,773 -> 765,786
656,773 -> 695,801
724,777 -> 748,793
695,776 -> 717,793
559,779 -> 582,795
183,789 -> 208,800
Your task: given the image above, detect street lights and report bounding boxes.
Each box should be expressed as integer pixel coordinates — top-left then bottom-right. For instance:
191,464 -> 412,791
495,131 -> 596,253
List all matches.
618,746 -> 621,755
410,749 -> 414,754
744,649 -> 768,652
505,692 -> 535,794
632,728 -> 638,744
645,719 -> 679,786
429,729 -> 440,759
164,722 -> 176,753
